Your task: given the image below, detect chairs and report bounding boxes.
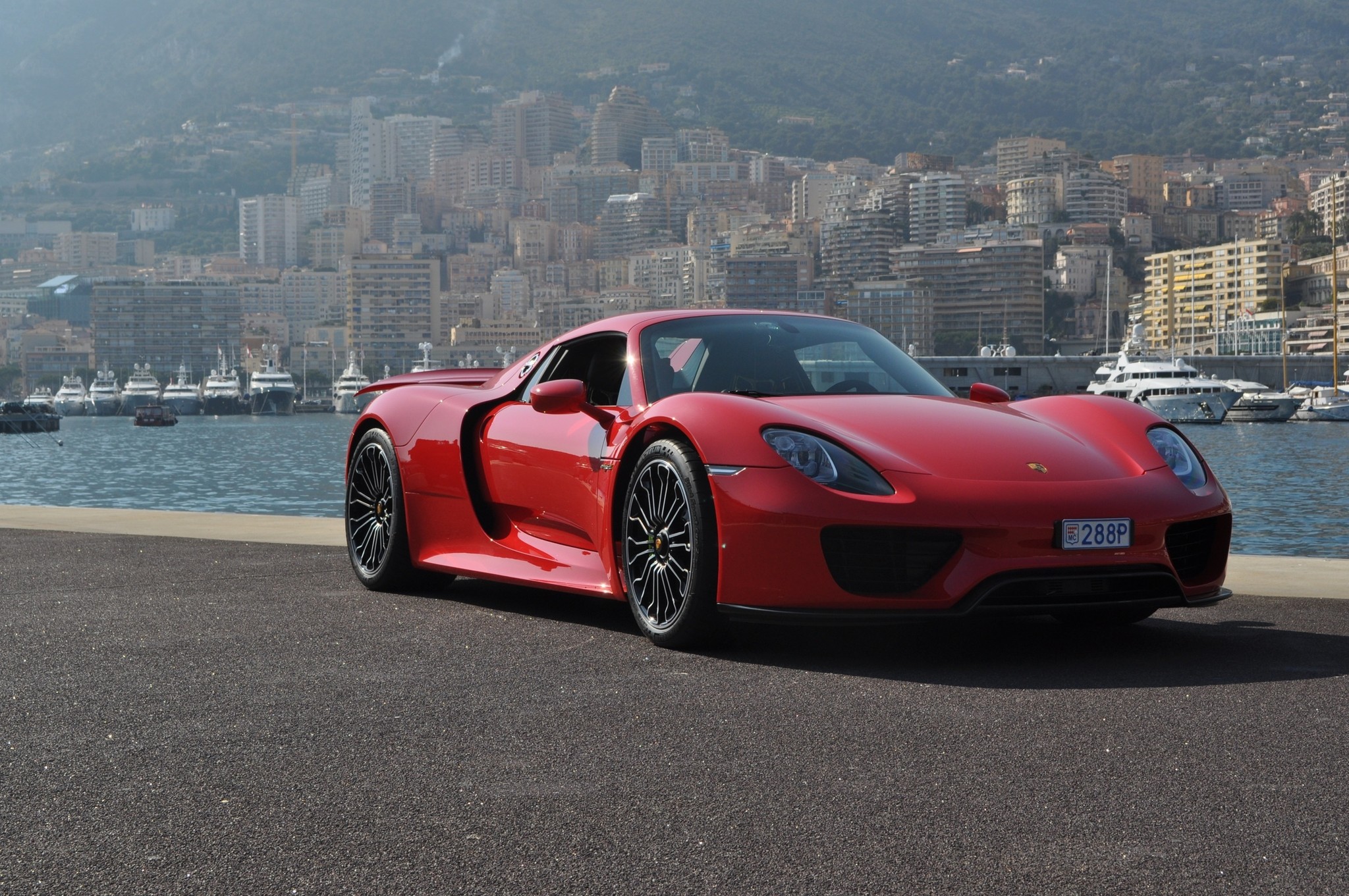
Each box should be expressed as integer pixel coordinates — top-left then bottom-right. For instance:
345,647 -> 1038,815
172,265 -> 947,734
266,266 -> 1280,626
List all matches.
581,353 -> 832,402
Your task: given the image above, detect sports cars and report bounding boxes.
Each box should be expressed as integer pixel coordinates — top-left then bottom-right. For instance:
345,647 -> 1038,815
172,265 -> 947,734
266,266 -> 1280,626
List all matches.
342,308 -> 1233,656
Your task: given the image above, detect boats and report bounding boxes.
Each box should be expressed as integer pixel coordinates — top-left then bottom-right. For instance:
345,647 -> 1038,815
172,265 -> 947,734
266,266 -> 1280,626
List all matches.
203,343 -> 243,415
334,350 -> 376,413
135,404 -> 178,426
248,343 -> 295,415
1199,368 -> 1308,422
88,363 -> 122,416
411,341 -> 516,372
1086,322 -> 1245,424
23,385 -> 55,408
53,366 -> 87,416
163,360 -> 203,416
120,353 -> 163,415
375,364 -> 392,397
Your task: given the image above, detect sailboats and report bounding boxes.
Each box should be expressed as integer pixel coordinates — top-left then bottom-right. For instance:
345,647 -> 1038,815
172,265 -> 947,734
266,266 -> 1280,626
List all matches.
1279,170 -> 1349,422
295,343 -> 332,412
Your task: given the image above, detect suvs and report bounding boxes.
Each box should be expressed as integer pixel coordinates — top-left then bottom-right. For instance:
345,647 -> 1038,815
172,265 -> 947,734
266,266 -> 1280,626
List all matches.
0,401 -> 33,415
